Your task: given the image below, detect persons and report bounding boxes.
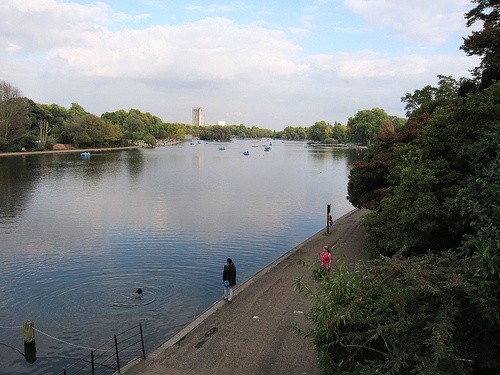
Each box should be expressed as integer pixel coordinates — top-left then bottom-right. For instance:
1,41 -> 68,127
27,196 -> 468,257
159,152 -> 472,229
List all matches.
320,246 -> 331,272
223,258 -> 236,303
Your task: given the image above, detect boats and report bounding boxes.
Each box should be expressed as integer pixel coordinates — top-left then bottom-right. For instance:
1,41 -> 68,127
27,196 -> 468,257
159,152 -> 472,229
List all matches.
243,151 -> 249,155
80,152 -> 91,156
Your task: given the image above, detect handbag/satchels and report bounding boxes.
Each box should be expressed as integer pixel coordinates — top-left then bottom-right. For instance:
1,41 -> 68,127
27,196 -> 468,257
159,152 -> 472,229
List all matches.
222,280 -> 229,287
